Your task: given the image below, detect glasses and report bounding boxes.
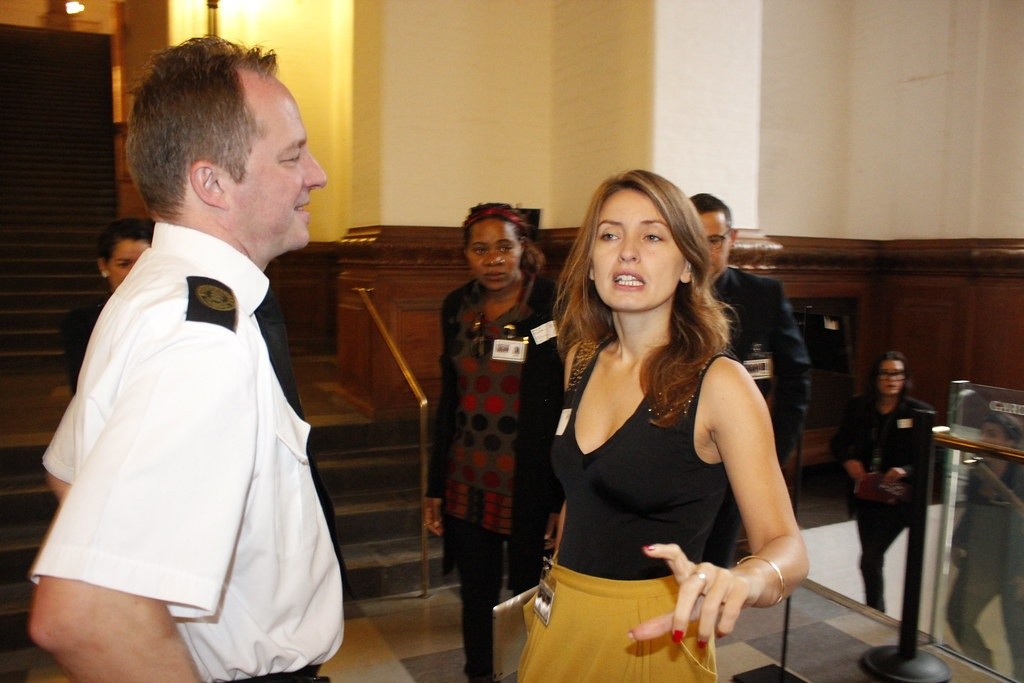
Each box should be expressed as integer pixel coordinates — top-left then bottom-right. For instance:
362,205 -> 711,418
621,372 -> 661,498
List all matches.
878,371 -> 907,381
704,228 -> 732,249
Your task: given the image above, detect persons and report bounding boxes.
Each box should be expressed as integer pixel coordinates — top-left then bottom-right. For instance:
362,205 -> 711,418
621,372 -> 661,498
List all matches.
27,35 -> 346,683
516,169 -> 810,683
942,410 -> 1024,683
834,351 -> 935,611
689,193 -> 811,568
57,217 -> 156,397
423,202 -> 565,683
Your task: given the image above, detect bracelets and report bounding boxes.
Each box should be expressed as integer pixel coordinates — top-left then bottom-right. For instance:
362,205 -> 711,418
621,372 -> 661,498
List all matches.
736,555 -> 785,609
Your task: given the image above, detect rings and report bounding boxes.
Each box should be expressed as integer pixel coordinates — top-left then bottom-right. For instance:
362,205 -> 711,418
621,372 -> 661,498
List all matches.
692,571 -> 708,582
